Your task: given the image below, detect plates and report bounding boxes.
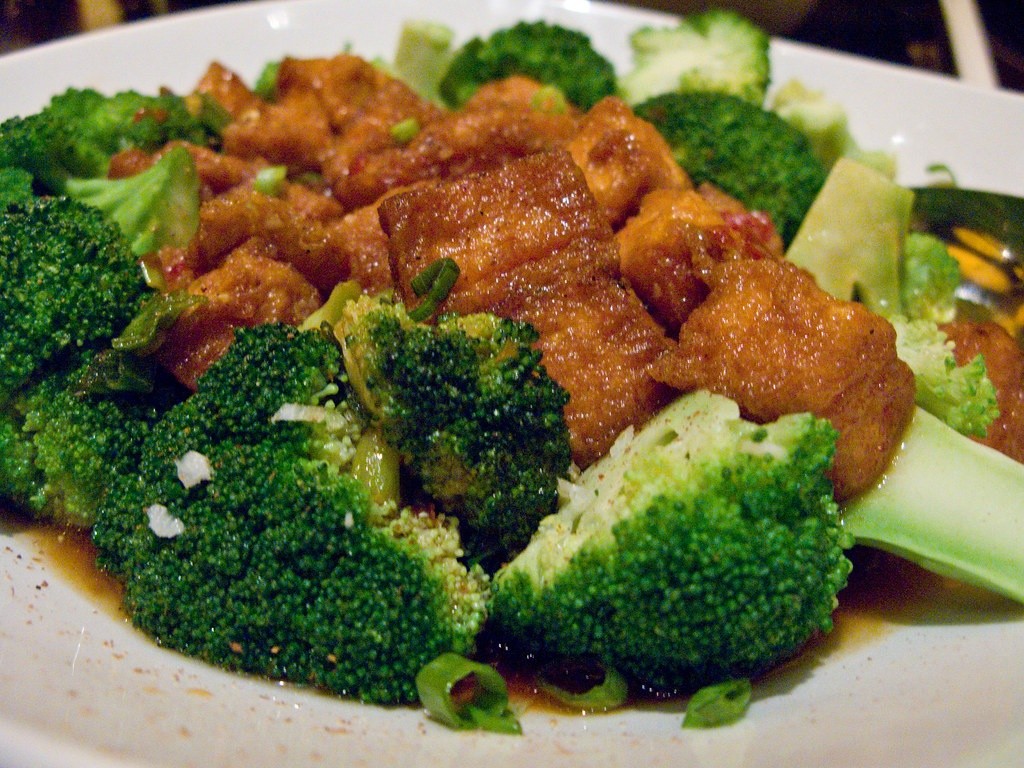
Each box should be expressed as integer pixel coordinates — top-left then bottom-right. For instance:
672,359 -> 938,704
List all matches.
0,0 -> 1024,768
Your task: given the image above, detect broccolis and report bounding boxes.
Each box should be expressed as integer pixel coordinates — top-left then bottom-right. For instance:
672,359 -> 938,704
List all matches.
0,8 -> 997,704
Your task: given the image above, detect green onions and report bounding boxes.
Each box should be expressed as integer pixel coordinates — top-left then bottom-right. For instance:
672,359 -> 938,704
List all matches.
413,654 -> 751,735
